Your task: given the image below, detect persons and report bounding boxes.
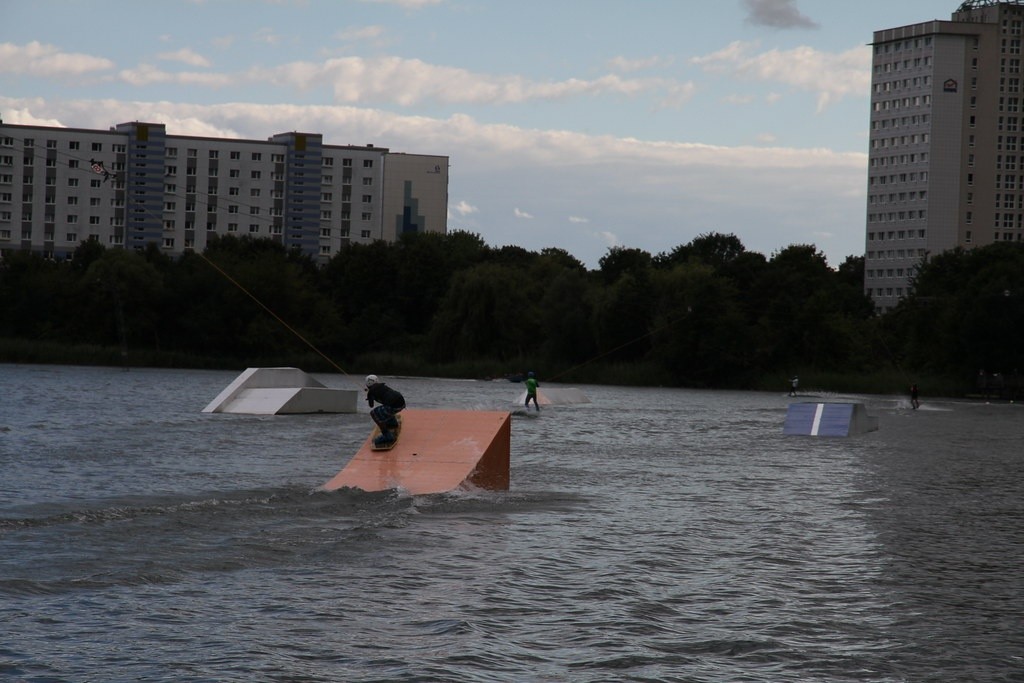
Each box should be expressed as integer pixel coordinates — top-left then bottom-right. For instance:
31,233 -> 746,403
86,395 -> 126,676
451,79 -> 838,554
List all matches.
525,372 -> 540,411
788,376 -> 798,396
365,375 -> 406,444
911,384 -> 919,409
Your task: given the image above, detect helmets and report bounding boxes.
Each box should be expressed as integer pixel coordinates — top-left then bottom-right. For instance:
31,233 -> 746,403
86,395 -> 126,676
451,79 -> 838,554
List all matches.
529,372 -> 533,377
365,374 -> 378,386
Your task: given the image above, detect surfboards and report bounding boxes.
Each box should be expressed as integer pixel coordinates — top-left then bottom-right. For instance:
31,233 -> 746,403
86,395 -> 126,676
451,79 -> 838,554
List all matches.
371,414 -> 402,451
